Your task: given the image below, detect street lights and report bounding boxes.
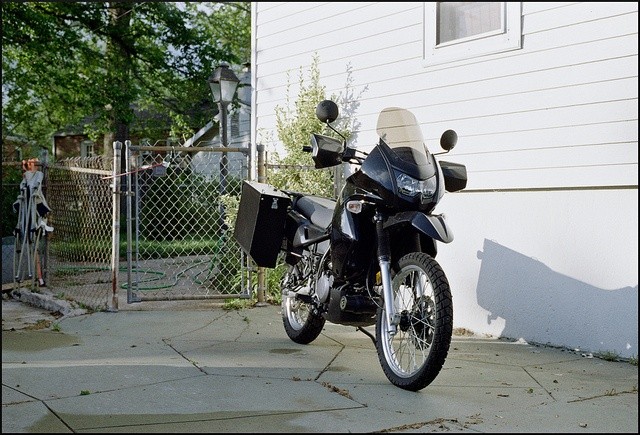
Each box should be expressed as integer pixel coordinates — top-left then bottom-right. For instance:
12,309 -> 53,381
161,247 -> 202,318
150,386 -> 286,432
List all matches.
208,64 -> 241,236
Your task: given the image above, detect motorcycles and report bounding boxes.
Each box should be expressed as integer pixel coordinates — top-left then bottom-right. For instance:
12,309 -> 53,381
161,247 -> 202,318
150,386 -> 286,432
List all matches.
233,100 -> 467,391
